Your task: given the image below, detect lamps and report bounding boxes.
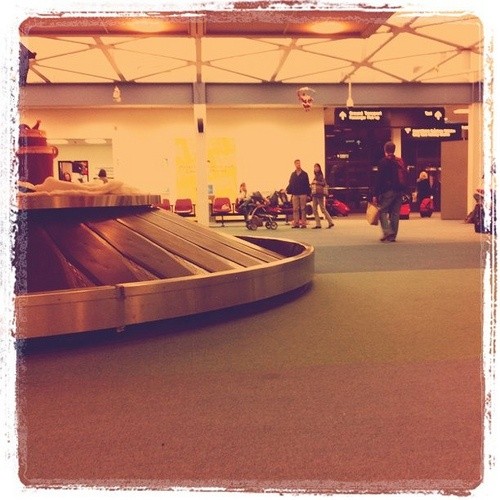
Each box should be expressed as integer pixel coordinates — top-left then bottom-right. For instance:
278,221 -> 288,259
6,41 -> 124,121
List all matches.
345,79 -> 354,110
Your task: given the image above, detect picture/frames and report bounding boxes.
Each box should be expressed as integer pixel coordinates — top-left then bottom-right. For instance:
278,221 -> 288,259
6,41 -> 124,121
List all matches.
58,160 -> 89,183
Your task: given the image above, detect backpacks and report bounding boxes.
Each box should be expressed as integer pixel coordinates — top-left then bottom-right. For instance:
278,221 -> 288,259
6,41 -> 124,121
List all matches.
394,158 -> 410,190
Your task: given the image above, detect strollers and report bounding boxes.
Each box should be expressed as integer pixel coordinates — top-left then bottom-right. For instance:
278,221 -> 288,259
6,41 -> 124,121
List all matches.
239,190 -> 285,231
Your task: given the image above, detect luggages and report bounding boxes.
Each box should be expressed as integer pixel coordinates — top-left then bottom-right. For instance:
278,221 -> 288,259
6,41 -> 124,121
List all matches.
329,199 -> 351,216
420,198 -> 434,217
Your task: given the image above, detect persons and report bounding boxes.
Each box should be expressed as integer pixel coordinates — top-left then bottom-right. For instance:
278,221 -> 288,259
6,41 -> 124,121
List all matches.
238,183 -> 247,205
417,171 -> 432,202
64,172 -> 71,181
310,163 -> 334,229
287,159 -> 311,229
372,141 -> 405,243
97,169 -> 108,184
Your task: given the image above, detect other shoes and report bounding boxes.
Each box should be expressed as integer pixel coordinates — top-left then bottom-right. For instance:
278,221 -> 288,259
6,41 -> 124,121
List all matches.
300,225 -> 306,228
325,224 -> 334,229
292,226 -> 299,228
312,226 -> 321,229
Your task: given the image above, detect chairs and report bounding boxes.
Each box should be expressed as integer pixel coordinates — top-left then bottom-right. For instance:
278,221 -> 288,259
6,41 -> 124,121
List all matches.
161,198 -> 173,213
174,199 -> 196,218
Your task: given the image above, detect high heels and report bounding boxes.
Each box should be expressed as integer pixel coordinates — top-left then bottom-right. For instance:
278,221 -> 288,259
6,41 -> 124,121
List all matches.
381,234 -> 395,241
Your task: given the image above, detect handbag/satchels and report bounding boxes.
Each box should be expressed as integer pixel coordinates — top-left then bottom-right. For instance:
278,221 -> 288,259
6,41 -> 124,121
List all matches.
365,202 -> 381,225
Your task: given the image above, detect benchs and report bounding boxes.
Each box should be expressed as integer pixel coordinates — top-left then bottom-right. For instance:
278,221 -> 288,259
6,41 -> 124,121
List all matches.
210,195 -> 313,227
